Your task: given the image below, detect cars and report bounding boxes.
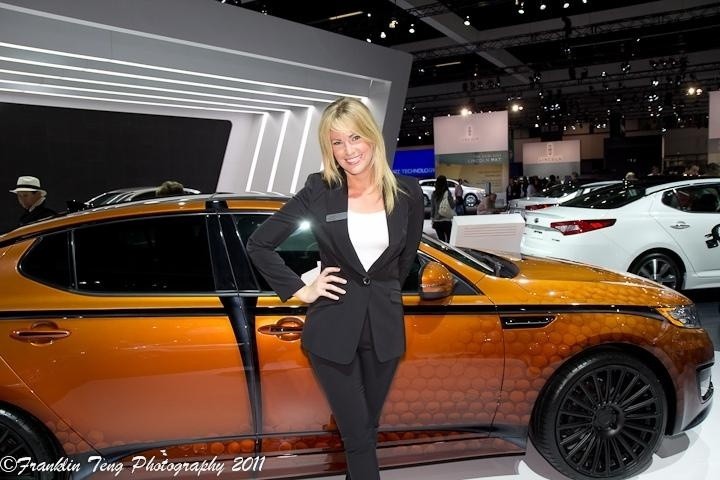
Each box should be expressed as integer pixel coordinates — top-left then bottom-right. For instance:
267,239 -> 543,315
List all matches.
0,172 -> 720,479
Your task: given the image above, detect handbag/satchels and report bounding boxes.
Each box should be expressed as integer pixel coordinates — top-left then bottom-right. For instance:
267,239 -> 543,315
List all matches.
439,190 -> 457,219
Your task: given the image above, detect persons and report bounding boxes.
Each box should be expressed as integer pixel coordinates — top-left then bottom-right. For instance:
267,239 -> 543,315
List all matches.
7,175 -> 61,228
428,175 -> 456,243
476,193 -> 504,215
454,178 -> 468,216
245,95 -> 426,479
506,161 -> 720,205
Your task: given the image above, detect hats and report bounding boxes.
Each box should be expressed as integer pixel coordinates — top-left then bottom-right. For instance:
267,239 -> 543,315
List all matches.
9,175 -> 47,196
